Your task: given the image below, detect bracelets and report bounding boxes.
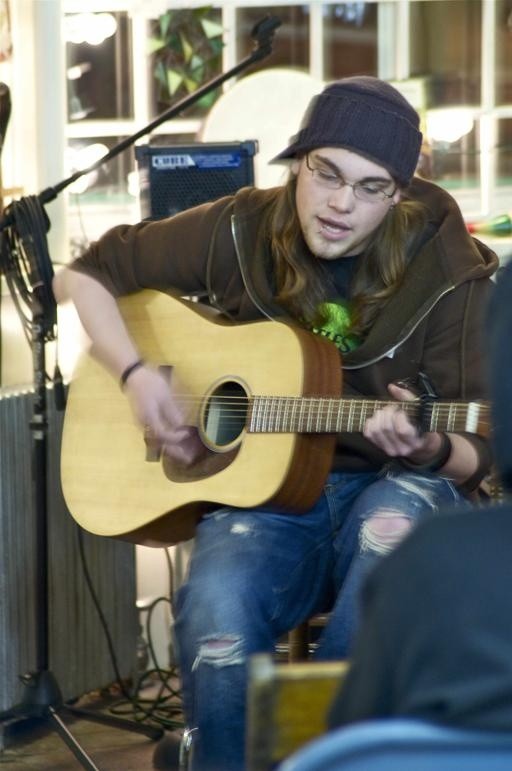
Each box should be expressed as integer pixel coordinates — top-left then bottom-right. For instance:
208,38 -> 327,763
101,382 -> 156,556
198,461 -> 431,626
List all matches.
118,359 -> 142,393
400,430 -> 452,474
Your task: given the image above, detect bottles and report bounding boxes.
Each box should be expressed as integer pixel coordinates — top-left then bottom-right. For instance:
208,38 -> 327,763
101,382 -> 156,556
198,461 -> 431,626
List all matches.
463,213 -> 512,239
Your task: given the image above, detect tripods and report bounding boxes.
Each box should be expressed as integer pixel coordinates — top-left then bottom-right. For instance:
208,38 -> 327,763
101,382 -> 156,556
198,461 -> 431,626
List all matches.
0,199 -> 167,771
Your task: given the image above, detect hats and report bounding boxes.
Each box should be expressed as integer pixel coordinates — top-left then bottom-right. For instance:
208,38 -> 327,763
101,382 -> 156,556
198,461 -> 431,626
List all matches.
266,75 -> 424,191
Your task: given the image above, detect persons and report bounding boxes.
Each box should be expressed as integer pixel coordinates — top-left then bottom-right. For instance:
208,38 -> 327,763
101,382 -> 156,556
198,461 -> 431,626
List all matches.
49,72 -> 498,770
325,257 -> 511,737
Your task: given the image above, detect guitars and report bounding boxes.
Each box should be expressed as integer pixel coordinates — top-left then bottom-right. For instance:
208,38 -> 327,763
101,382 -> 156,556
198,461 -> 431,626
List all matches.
61,287 -> 495,545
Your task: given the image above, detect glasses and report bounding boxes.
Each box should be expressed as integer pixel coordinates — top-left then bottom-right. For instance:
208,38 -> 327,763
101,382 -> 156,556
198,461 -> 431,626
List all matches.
303,151 -> 399,203
